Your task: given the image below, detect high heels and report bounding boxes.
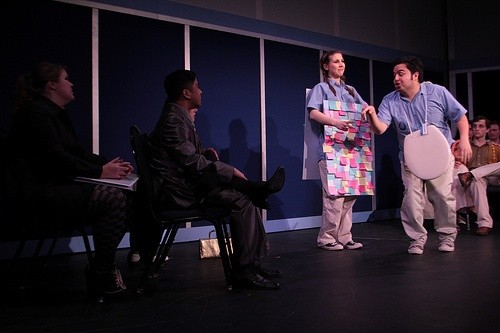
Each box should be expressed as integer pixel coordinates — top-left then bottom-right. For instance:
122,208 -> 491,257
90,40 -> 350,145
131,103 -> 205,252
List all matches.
244,166 -> 285,210
84,262 -> 134,298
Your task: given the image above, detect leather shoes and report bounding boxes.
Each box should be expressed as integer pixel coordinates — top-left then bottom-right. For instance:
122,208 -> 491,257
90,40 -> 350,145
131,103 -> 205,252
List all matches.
248,261 -> 284,278
232,271 -> 281,291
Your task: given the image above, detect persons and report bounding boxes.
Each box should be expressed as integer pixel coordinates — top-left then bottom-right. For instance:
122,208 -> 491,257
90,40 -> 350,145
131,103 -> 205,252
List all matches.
306,50 -> 375,251
423,119 -> 500,235
146,67 -> 286,291
359,55 -> 472,254
0,60 -> 135,300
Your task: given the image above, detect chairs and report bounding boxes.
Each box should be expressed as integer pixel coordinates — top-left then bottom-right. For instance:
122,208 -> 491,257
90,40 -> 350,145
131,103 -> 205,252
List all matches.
1,138 -> 240,319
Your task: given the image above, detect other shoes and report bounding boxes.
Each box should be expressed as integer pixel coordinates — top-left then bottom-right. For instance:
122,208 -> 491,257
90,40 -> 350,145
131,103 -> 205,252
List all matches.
344,240 -> 363,250
438,238 -> 457,252
457,172 -> 471,187
475,227 -> 493,235
320,243 -> 342,250
408,235 -> 432,254
